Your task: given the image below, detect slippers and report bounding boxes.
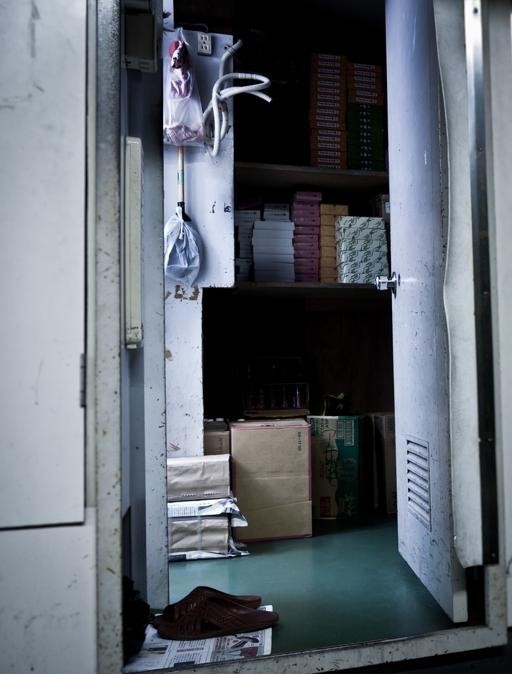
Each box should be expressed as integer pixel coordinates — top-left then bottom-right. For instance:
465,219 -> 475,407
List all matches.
157,591 -> 279,641
150,586 -> 262,630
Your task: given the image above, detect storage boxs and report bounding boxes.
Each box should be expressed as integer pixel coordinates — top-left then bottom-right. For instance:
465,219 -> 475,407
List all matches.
370,412 -> 395,516
230,409 -> 314,545
308,415 -> 370,522
204,421 -> 230,455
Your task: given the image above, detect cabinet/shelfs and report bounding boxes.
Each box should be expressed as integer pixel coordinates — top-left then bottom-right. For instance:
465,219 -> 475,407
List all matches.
233,161 -> 389,288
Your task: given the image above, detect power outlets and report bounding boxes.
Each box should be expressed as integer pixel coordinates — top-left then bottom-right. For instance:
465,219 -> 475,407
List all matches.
197,32 -> 213,56
